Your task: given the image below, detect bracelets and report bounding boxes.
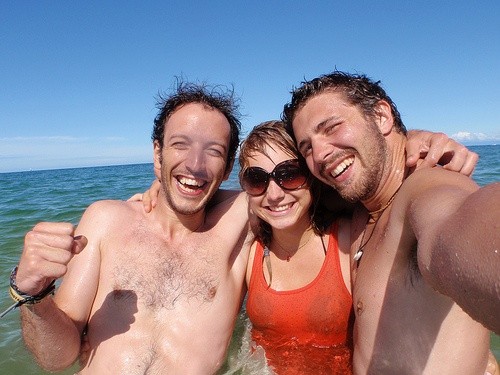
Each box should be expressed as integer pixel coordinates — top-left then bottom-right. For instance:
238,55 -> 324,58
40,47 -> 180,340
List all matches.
0,262 -> 55,320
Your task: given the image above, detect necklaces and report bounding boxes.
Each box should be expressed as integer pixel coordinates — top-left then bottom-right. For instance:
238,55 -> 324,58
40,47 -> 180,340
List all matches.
274,233 -> 319,262
354,168 -> 411,262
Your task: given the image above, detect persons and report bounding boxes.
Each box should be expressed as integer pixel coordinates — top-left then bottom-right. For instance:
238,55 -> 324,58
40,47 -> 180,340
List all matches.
122,68 -> 500,375
79,120 -> 354,375
0,76 -> 479,375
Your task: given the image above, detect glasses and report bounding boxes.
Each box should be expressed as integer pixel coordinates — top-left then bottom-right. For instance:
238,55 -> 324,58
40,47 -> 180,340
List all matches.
239,159 -> 308,196
